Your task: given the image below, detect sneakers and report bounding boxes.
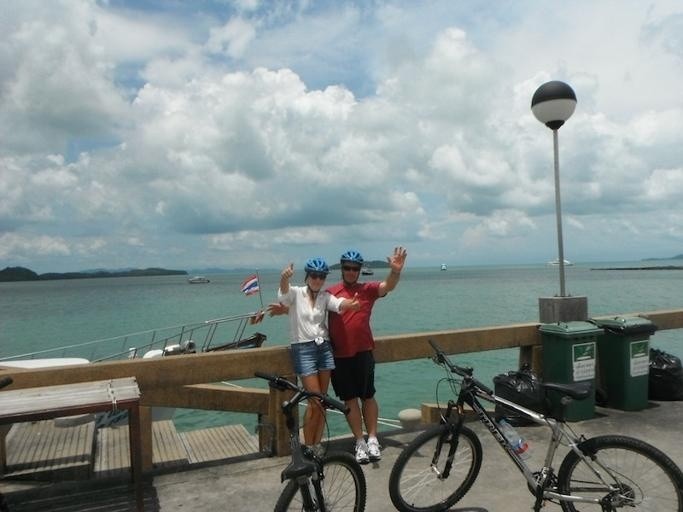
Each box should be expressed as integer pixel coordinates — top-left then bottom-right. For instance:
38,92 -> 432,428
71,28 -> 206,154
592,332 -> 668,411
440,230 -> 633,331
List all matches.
352,439 -> 382,464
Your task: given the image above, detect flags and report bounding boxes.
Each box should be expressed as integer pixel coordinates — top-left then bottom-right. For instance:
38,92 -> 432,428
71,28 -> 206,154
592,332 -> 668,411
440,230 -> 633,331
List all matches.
240,274 -> 260,297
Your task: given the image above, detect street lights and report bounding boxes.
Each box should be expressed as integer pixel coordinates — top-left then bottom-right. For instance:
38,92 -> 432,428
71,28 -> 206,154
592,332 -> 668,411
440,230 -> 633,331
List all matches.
531,81 -> 579,299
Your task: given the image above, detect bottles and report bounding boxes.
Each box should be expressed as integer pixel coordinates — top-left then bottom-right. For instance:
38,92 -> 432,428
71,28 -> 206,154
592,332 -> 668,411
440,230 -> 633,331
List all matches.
500,419 -> 532,461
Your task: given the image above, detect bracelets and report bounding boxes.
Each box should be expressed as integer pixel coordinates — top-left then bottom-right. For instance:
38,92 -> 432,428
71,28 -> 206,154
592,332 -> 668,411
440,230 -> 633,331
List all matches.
391,270 -> 400,276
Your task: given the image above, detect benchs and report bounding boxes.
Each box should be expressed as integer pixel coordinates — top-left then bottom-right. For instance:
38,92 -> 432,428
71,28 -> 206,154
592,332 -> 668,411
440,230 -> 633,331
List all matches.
1,376 -> 146,511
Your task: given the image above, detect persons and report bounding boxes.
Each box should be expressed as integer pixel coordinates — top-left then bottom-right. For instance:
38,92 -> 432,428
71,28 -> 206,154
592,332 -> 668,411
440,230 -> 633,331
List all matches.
277,256 -> 360,458
265,245 -> 407,463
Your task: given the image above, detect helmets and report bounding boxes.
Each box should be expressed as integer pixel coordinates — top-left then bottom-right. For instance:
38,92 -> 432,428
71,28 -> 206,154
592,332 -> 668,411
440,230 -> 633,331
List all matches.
340,251 -> 364,265
304,255 -> 330,275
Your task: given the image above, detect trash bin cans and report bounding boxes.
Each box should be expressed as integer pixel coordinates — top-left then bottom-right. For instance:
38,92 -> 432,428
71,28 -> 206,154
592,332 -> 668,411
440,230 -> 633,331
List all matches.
538,319 -> 605,422
592,316 -> 657,411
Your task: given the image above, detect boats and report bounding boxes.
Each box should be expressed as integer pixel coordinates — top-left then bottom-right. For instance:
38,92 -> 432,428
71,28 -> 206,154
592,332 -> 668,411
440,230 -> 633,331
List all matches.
362,270 -> 374,275
0,311 -> 267,427
441,264 -> 447,270
188,276 -> 210,284
546,257 -> 574,267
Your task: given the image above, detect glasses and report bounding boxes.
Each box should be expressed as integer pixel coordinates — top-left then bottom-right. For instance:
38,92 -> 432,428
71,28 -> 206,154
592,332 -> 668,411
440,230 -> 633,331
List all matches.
309,273 -> 326,280
343,267 -> 359,272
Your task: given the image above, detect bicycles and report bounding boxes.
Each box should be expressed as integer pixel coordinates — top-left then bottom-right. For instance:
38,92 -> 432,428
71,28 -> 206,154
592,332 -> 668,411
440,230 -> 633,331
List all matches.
389,338 -> 683,511
253,372 -> 367,512
